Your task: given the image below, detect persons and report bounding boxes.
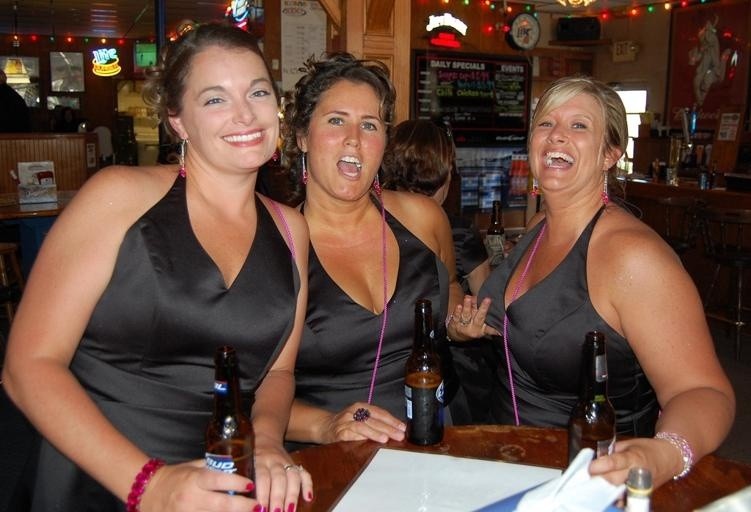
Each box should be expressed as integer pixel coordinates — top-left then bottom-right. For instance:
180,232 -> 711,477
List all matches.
475,71 -> 739,510
375,116 -> 493,294
0,19 -> 315,512
284,51 -> 505,445
1,62 -> 85,133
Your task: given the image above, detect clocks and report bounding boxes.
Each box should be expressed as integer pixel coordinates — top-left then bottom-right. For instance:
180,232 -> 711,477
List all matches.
506,12 -> 542,53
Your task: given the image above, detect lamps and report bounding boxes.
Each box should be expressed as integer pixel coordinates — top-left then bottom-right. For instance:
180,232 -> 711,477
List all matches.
608,40 -> 641,64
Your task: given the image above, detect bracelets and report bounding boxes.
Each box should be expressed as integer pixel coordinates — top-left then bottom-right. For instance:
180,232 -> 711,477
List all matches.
652,431 -> 693,478
121,460 -> 167,511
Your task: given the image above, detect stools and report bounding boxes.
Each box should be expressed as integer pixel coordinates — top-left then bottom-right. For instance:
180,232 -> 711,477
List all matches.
1,241 -> 27,335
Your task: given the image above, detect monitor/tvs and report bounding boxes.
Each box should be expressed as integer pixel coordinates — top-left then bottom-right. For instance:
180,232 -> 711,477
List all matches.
133,39 -> 157,73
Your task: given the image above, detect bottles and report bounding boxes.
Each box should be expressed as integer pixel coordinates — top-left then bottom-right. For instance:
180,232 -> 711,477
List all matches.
404,300 -> 444,447
488,201 -> 507,266
509,149 -> 529,200
690,102 -> 698,134
568,332 -> 618,465
698,164 -> 728,191
696,143 -> 712,166
205,345 -> 261,499
649,158 -> 673,182
626,467 -> 655,510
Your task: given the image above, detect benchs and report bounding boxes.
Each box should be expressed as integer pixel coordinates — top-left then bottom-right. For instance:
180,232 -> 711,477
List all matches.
1,131 -> 91,198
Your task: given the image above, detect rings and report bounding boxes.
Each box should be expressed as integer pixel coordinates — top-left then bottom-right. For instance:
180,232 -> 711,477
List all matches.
460,311 -> 473,324
283,462 -> 302,476
353,405 -> 372,423
